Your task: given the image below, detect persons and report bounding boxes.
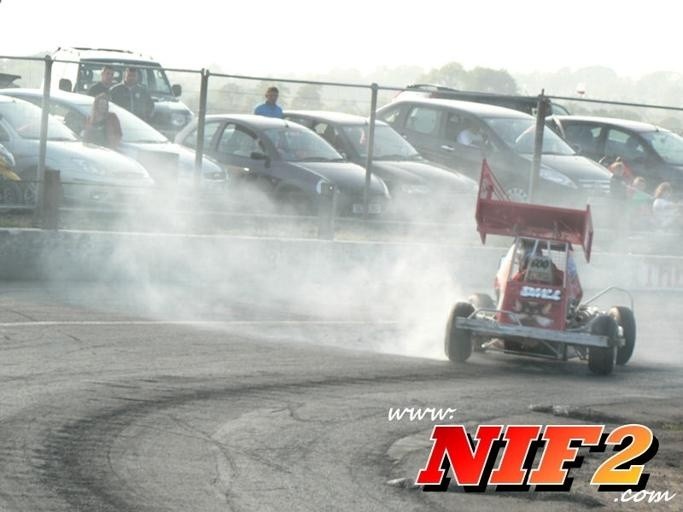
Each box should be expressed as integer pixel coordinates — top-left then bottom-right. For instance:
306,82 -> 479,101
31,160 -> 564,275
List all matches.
654,181 -> 682,222
84,64 -> 116,100
631,176 -> 647,200
108,68 -> 153,124
609,162 -> 628,197
82,92 -> 123,151
251,87 -> 284,151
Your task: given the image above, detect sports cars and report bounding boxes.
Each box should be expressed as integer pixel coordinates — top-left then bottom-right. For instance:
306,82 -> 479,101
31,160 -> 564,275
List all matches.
439,152 -> 642,372
